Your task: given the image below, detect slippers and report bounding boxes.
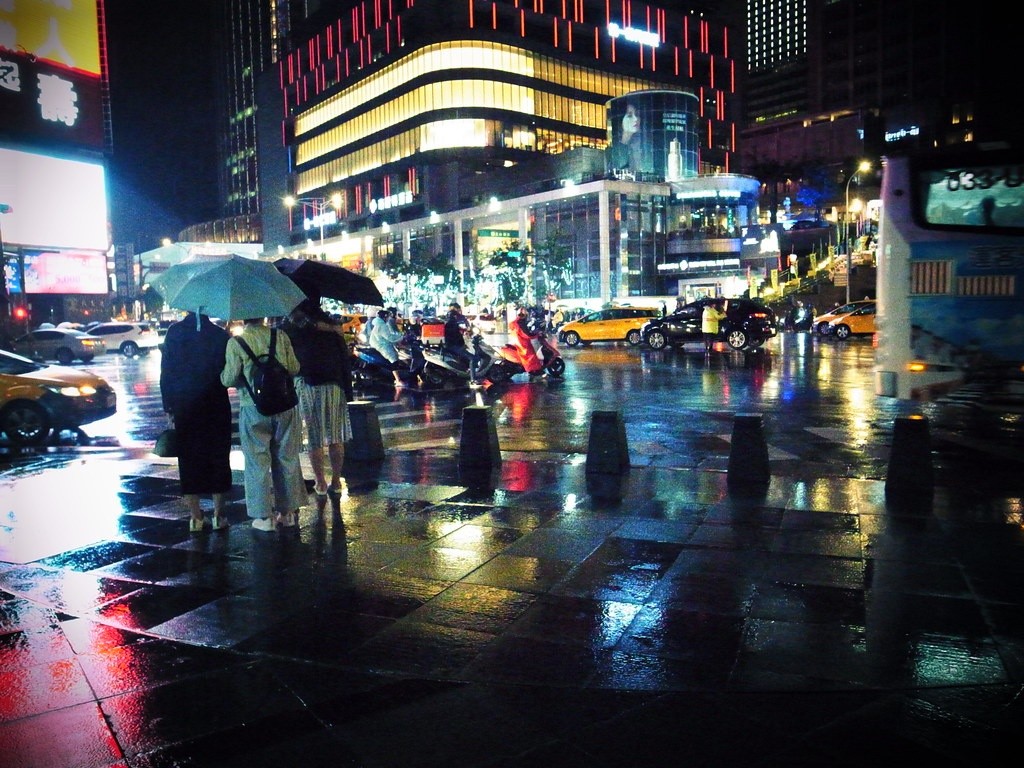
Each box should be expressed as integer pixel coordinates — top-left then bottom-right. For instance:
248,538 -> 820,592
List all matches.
313,482 -> 327,495
328,483 -> 341,492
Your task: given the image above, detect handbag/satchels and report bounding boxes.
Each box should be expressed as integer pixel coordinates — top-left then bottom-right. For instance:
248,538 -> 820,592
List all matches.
154,418 -> 178,457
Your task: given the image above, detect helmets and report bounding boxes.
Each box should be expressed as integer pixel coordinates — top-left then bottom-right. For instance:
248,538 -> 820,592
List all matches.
447,310 -> 460,318
517,307 -> 528,320
387,307 -> 397,315
449,303 -> 460,310
412,310 -> 424,317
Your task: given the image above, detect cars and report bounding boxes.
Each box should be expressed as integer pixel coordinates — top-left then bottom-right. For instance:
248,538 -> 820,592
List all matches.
326,303 -> 496,344
10,328 -> 106,365
770,300 -> 876,340
0,349 -> 117,445
556,307 -> 660,347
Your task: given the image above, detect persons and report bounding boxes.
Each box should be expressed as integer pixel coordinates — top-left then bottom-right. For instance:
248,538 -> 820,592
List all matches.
662,303 -> 666,317
527,308 -> 537,328
835,302 -> 839,307
513,307 -> 549,379
702,300 -> 726,355
785,301 -> 817,332
219,318 -> 311,531
387,307 -> 399,331
278,298 -> 352,495
552,308 -> 563,327
445,303 -> 478,368
677,300 -> 686,309
159,311 -> 235,531
366,317 -> 375,336
608,100 -> 641,169
370,310 -> 407,387
978,197 -> 995,224
545,310 -> 550,328
565,308 -> 585,322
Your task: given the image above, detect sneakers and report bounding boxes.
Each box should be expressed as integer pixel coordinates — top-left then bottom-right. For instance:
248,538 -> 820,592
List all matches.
190,510 -> 209,532
211,514 -> 227,530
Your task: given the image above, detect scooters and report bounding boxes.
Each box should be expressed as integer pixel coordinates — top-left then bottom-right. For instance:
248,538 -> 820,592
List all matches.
349,330 -> 566,390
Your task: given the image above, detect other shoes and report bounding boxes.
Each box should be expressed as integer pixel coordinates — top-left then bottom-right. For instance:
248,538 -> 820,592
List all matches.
394,380 -> 409,387
535,373 -> 548,379
253,515 -> 275,531
277,513 -> 295,525
470,380 -> 482,388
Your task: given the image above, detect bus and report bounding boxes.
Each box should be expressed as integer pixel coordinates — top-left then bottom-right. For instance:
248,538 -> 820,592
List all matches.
877,107 -> 1024,449
548,294 -> 686,333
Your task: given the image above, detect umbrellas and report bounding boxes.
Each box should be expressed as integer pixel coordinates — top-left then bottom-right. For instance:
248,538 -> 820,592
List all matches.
272,257 -> 384,308
151,254 -> 308,322
676,298 -> 685,301
658,300 -> 667,303
39,321 -> 101,328
558,305 -> 567,307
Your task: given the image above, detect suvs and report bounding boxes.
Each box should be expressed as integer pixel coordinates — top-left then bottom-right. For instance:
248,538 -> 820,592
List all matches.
640,297 -> 779,350
86,323 -> 159,358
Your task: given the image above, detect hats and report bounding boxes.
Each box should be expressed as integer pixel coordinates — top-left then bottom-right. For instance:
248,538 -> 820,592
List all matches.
378,310 -> 389,319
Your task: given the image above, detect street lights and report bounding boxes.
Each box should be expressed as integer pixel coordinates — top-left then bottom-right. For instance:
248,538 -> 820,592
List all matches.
281,192 -> 343,252
846,161 -> 871,305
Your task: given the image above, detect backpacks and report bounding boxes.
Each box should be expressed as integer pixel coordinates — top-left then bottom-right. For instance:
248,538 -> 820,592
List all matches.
235,328 -> 298,416
